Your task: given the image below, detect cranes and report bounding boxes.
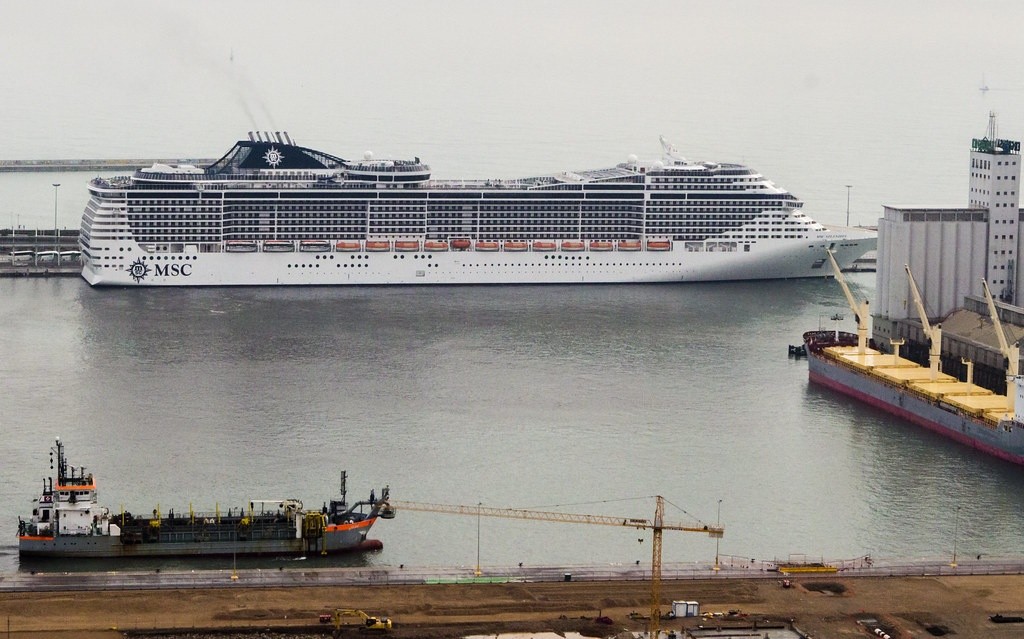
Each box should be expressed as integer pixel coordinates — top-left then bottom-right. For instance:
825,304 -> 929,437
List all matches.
382,496 -> 726,639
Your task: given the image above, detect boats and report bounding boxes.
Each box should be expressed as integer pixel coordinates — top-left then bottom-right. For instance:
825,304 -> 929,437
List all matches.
801,247 -> 1023,465
14,432 -> 396,555
776,560 -> 840,575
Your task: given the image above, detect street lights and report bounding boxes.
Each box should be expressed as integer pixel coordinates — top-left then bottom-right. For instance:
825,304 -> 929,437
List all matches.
952,505 -> 963,568
846,184 -> 853,227
53,183 -> 61,236
475,502 -> 485,577
713,498 -> 723,575
232,505 -> 239,575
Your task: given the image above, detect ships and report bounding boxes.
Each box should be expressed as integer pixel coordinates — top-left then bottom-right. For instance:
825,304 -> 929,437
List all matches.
77,130 -> 880,286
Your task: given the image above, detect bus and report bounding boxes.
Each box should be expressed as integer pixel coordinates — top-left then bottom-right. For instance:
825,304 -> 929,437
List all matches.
57,251 -> 83,262
34,251 -> 58,265
8,250 -> 36,262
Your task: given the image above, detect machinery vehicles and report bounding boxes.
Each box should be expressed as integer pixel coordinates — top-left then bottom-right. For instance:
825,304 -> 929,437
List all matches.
331,607 -> 393,635
